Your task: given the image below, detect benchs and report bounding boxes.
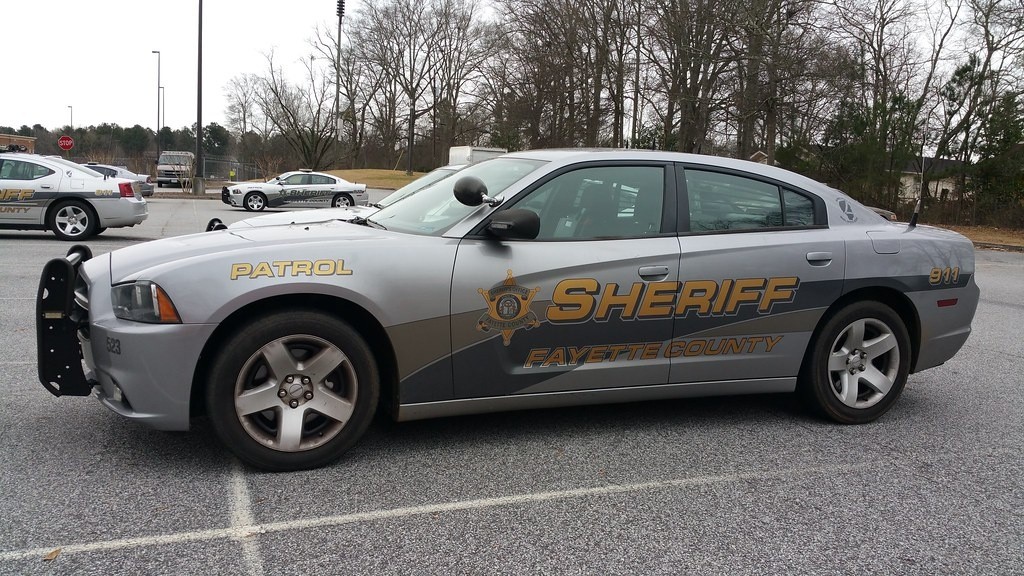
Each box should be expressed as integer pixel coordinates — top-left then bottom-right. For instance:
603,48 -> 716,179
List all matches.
692,203 -> 782,231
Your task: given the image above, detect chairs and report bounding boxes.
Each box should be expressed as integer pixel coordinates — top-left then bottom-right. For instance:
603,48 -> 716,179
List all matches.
622,188 -> 664,238
551,184 -> 615,241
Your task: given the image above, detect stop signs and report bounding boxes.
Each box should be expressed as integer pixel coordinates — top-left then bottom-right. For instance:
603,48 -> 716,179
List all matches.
57,135 -> 74,151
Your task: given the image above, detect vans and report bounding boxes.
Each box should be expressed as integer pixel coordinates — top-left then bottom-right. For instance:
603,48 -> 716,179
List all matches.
155,151 -> 195,188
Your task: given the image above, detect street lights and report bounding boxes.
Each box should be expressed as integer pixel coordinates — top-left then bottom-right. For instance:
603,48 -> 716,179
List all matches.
152,51 -> 160,160
160,87 -> 165,148
433,49 -> 445,160
68,106 -> 72,132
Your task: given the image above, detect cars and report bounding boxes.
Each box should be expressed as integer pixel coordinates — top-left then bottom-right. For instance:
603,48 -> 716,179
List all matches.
221,167 -> 370,210
0,145 -> 150,240
35,145 -> 983,473
864,206 -> 898,222
79,162 -> 154,196
206,163 -> 639,231
